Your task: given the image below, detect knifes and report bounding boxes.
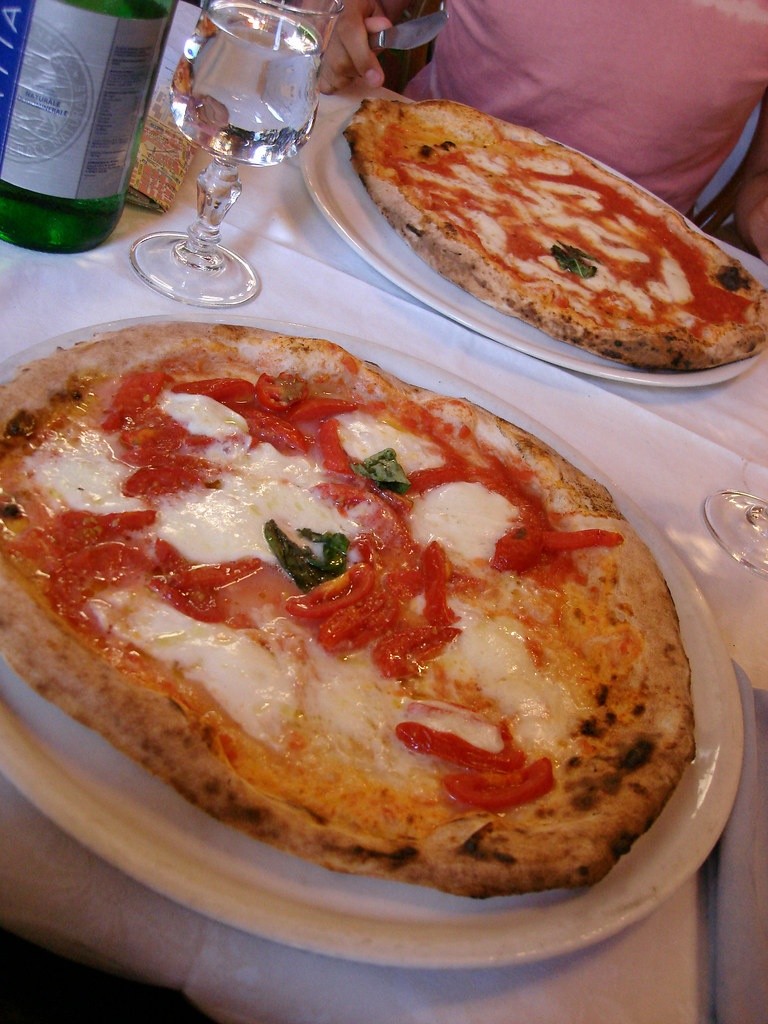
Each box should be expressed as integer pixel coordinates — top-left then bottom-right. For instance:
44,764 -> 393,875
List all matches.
370,10 -> 448,50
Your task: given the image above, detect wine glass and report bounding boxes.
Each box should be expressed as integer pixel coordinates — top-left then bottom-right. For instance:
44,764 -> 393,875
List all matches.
131,0 -> 346,309
704,489 -> 768,581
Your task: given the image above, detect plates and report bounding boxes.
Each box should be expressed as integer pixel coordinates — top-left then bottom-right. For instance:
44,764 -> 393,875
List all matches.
0,316 -> 743,969
299,104 -> 765,385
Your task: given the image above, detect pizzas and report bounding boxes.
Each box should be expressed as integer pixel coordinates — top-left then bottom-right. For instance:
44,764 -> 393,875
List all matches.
344,98 -> 768,373
0,323 -> 696,898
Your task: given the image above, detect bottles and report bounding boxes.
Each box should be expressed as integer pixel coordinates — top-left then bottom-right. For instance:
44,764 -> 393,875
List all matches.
0,0 -> 179,253
